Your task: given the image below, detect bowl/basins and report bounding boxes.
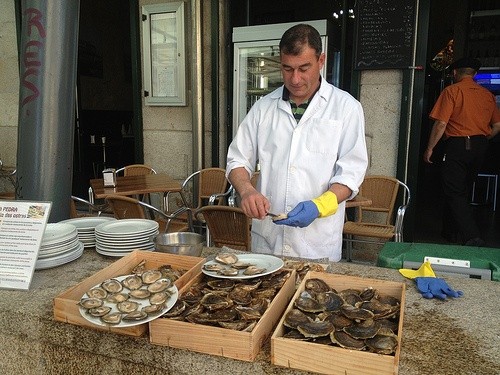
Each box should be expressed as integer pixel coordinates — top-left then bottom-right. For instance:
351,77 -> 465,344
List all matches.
152,231 -> 207,257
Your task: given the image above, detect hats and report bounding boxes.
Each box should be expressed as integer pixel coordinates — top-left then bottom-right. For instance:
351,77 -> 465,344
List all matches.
451,53 -> 483,72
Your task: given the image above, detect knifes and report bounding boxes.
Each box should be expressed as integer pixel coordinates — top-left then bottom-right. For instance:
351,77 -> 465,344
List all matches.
266,212 -> 279,218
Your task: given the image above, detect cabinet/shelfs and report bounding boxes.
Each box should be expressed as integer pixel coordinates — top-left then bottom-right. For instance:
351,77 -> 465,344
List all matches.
467,9 -> 500,69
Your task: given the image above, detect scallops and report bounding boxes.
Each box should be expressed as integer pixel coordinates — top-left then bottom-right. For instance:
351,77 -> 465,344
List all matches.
80,252 -> 400,355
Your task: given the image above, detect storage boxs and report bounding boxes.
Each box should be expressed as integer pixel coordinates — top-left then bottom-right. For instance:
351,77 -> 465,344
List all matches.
269,272 -> 406,375
149,268 -> 297,363
52,250 -> 207,337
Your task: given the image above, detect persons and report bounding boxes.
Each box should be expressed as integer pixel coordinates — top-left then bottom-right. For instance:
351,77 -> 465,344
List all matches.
223,24 -> 368,262
423,57 -> 500,245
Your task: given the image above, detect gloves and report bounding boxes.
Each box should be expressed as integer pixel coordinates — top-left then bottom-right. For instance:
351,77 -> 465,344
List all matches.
399,261 -> 463,299
272,190 -> 339,227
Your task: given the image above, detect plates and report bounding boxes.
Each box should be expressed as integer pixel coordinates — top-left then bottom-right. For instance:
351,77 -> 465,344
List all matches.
34,217 -> 159,270
79,274 -> 179,327
201,254 -> 284,279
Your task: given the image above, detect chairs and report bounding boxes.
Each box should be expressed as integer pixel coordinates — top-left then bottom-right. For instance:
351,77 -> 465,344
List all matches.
164,168 -> 233,248
194,206 -> 251,251
90,165 -> 156,207
70,195 -> 109,218
105,194 -> 194,235
228,172 -> 259,207
345,177 -> 410,261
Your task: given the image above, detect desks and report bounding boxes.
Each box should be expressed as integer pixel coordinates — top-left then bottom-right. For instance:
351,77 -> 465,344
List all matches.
90,173 -> 182,198
